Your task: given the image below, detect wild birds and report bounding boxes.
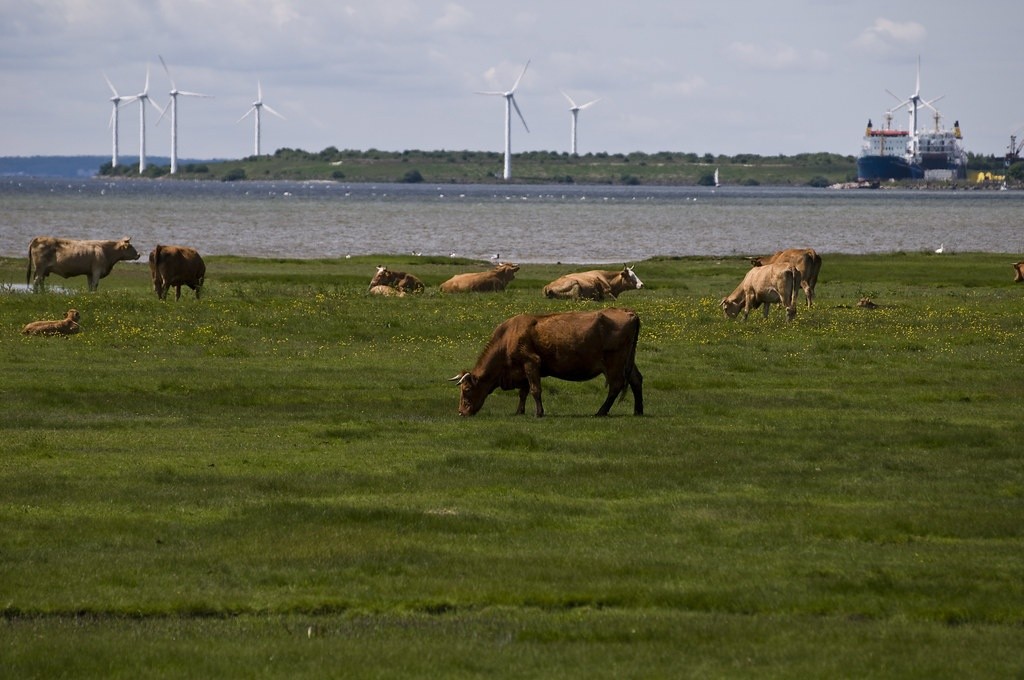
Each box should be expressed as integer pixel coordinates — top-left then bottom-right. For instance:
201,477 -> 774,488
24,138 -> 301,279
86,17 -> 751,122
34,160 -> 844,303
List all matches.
411,250 -> 422,256
448,252 -> 455,258
491,254 -> 500,259
345,252 -> 351,259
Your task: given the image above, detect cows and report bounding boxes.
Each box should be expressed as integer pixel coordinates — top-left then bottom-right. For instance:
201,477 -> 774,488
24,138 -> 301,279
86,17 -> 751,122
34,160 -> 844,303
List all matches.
25,235 -> 140,292
439,262 -> 521,294
750,247 -> 823,310
366,265 -> 425,298
149,244 -> 206,301
542,262 -> 644,302
20,309 -> 81,336
447,306 -> 644,418
719,262 -> 801,324
